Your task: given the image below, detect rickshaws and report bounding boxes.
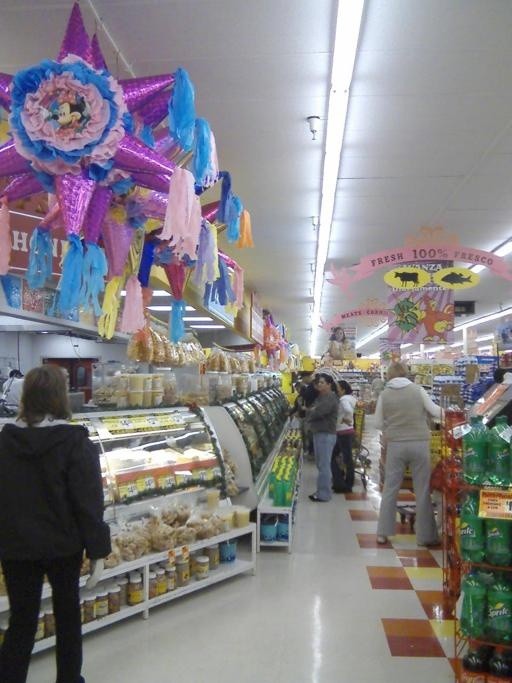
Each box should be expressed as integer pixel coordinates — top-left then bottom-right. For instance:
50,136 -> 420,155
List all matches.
396,488 -> 436,527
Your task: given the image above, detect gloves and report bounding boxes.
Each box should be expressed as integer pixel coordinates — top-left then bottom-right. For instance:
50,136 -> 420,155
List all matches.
85,559 -> 104,589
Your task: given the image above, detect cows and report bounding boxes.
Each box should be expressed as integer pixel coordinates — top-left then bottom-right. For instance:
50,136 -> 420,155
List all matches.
393,270 -> 420,288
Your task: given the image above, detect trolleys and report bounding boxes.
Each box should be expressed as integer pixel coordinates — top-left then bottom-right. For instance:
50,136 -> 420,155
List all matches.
339,409 -> 371,489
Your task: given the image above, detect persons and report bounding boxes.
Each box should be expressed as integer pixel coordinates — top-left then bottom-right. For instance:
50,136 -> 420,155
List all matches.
329,327 -> 347,360
373,362 -> 445,546
3,370 -> 24,404
0,365 -> 111,683
285,372 -> 357,502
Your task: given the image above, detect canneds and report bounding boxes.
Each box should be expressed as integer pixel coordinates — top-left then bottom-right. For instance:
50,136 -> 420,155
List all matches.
112,373 -> 164,408
0,543 -> 220,647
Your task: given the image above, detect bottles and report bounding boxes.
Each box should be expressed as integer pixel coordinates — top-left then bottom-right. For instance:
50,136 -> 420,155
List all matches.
270,429 -> 303,507
456,489 -> 512,566
465,413 -> 511,484
461,569 -> 512,645
457,646 -> 512,683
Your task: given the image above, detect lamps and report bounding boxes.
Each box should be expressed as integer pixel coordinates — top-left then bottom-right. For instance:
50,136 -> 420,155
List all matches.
307,115 -> 320,141
311,216 -> 319,230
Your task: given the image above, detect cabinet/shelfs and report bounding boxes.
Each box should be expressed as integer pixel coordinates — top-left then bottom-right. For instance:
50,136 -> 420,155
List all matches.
4,405 -> 258,658
437,384 -> 512,682
200,386 -> 305,555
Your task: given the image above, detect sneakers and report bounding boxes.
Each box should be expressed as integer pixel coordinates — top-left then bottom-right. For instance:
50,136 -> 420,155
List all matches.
376,535 -> 387,544
309,493 -> 330,501
417,540 -> 440,546
